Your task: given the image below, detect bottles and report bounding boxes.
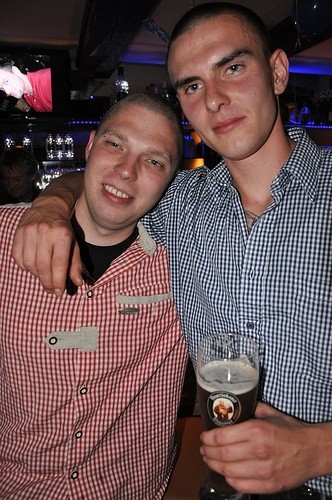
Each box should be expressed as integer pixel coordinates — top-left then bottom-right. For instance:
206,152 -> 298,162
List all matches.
54,133 -> 64,160
64,133 -> 74,159
113,64 -> 130,103
45,133 -> 55,161
151,84 -> 160,99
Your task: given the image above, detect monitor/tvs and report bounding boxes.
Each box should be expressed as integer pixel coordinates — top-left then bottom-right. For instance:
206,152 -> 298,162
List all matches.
0,47 -> 71,124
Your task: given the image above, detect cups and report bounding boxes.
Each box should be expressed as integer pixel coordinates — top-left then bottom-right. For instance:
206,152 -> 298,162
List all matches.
195,331 -> 260,500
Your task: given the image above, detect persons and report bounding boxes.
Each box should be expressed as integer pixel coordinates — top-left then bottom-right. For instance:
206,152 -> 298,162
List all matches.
11,0 -> 332,500
0,92 -> 209,500
